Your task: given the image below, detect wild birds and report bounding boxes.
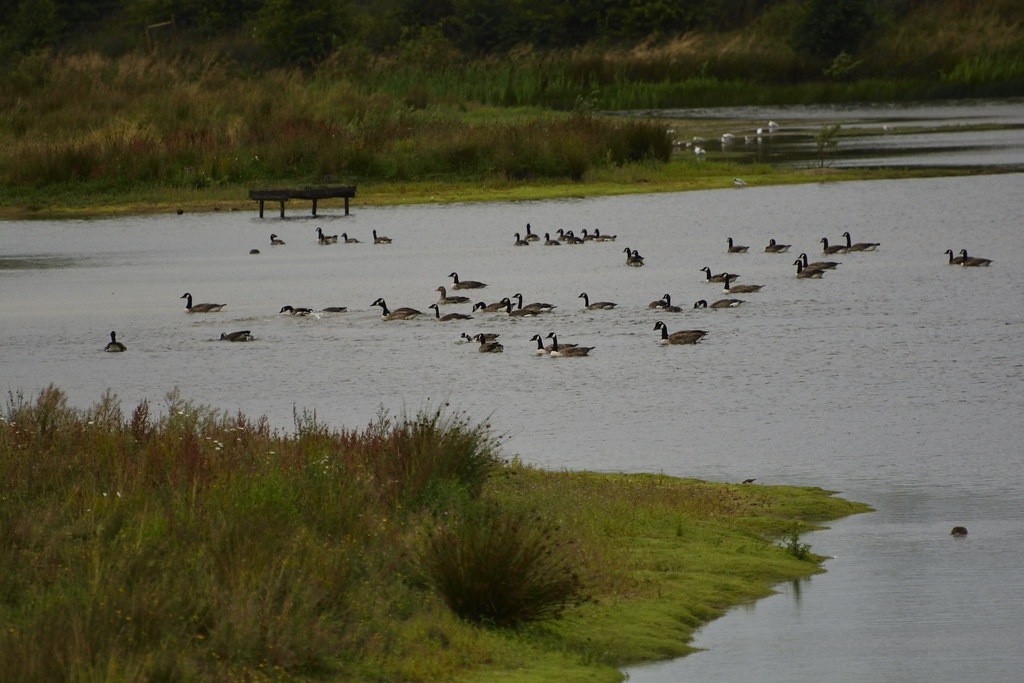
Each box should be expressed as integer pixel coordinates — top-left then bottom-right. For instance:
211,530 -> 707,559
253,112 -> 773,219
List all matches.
180,292 -> 227,314
666,120 -> 781,157
648,266 -> 767,312
513,222 -> 617,247
726,237 -> 749,253
278,271 -> 619,358
882,123 -> 897,135
623,247 -> 645,267
734,177 -> 748,189
765,239 -> 792,253
652,321 -> 709,345
793,232 -> 881,280
219,330 -> 255,342
104,331 -> 127,353
944,249 -> 995,268
249,226 -> 393,254
